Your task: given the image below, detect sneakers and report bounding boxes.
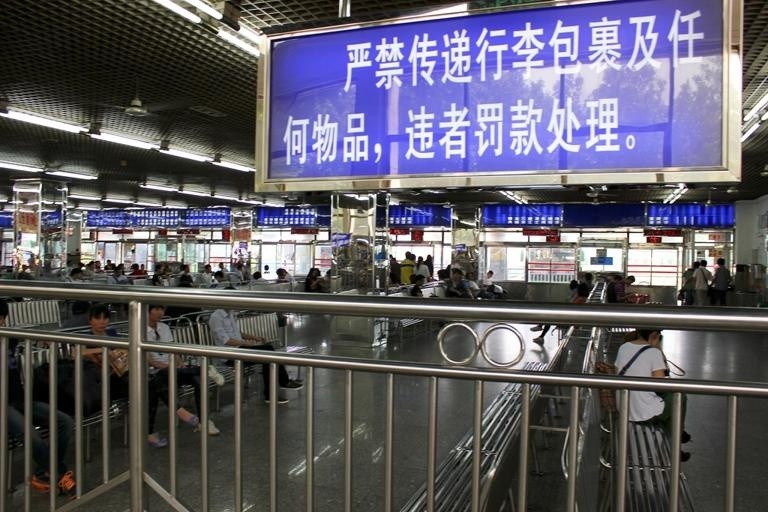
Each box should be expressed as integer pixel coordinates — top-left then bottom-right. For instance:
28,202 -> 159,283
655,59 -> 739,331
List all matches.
148,437 -> 166,447
281,382 -> 302,391
187,415 -> 198,425
534,337 -> 544,343
196,421 -> 219,435
208,365 -> 224,386
681,431 -> 690,442
266,395 -> 289,404
30,476 -> 52,491
680,451 -> 690,462
530,327 -> 542,331
61,475 -> 77,499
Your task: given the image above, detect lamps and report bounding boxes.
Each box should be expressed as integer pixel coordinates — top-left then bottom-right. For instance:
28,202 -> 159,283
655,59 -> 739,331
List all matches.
0,108 -> 286,213
156,0 -> 260,57
663,186 -> 688,204
742,94 -> 768,143
500,191 -> 527,204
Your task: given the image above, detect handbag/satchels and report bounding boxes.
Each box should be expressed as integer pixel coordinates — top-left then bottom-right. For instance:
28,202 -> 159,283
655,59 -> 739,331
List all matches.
110,349 -> 129,375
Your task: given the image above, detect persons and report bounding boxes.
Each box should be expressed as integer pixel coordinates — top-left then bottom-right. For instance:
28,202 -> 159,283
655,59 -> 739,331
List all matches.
682,257 -> 733,305
390,252 -> 508,327
2,251 -> 328,499
530,270 -> 595,342
606,273 -> 637,305
614,328 -> 692,462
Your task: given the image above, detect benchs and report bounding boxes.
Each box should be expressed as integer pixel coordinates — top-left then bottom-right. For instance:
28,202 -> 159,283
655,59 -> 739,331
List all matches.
551,282 -> 597,346
398,326 -> 578,510
6,269 -> 313,344
555,327 -> 698,510
9,311 -> 310,464
600,281 -> 662,353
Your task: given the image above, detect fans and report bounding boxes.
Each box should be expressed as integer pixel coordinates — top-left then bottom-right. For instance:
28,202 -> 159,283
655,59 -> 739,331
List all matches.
95,82 -> 192,117
